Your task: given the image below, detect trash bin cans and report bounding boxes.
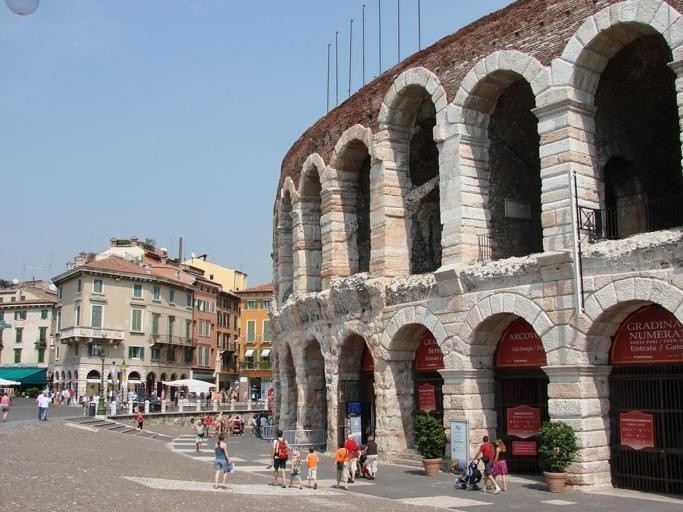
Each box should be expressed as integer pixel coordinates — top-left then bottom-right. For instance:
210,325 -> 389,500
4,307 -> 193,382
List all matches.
89,403 -> 95,416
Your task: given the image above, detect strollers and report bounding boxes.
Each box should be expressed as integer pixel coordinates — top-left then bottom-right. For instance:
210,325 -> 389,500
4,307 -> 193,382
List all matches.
354,450 -> 371,481
453,461 -> 480,492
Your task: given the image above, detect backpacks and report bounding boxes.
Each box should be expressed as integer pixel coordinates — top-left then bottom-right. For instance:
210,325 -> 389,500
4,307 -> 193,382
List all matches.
276,439 -> 288,461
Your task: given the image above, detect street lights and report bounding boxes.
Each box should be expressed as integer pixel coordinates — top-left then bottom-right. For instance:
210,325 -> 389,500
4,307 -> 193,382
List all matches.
93,333 -> 109,420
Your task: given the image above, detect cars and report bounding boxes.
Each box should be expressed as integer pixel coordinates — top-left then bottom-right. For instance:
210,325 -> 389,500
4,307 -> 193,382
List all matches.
108,392 -> 167,414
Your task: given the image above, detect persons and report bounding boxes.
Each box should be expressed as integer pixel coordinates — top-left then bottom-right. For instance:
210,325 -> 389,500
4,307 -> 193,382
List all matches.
137,406 -> 145,430
336,441 -> 350,490
490,438 -> 509,493
471,435 -> 502,496
0,392 -> 11,423
252,391 -> 260,401
82,388 -> 137,416
174,389 -> 179,406
250,410 -> 276,439
198,411 -> 245,436
36,390 -> 50,421
344,434 -> 358,483
272,430 -> 292,488
206,387 -> 239,403
265,387 -> 273,401
214,434 -> 232,489
193,419 -> 205,456
43,386 -> 75,407
288,450 -> 304,489
137,386 -> 166,401
306,446 -> 320,490
363,435 -> 377,480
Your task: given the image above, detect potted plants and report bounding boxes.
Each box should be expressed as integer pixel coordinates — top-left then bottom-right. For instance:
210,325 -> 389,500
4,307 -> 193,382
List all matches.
536,420 -> 583,492
412,413 -> 448,477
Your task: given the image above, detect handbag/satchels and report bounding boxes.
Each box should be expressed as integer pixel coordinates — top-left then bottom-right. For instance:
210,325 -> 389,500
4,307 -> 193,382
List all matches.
228,462 -> 234,474
483,455 -> 489,464
336,461 -> 344,470
